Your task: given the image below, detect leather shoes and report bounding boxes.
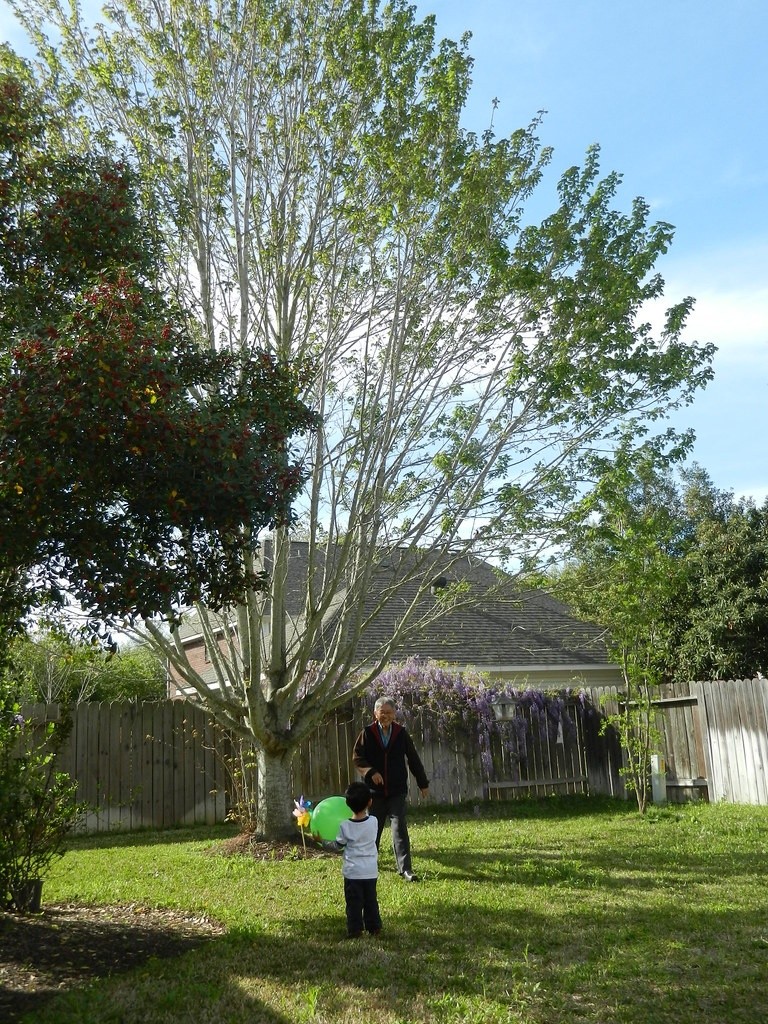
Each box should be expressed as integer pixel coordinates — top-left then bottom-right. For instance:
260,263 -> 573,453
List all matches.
402,870 -> 418,882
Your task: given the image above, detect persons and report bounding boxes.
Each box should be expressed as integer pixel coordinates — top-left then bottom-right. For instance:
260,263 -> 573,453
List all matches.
352,698 -> 428,881
312,782 -> 384,937
